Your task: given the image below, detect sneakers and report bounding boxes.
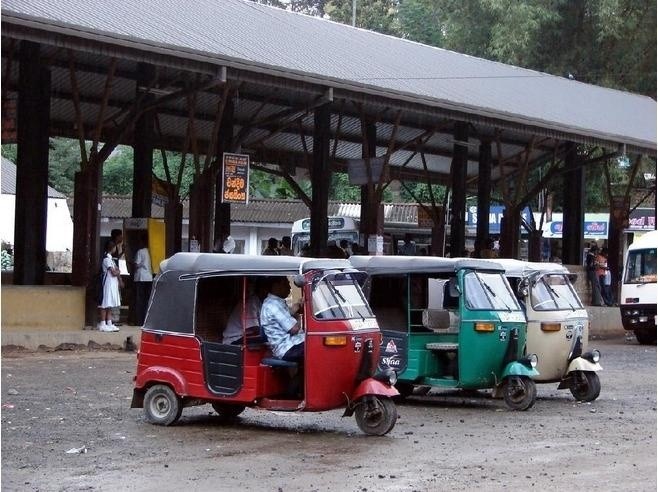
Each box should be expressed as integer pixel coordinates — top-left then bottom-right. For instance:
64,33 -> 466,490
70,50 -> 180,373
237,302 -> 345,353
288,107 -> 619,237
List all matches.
99,324 -> 120,332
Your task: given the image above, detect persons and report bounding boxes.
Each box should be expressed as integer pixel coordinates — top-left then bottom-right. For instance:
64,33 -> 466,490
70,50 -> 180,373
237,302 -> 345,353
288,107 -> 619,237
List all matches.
259,276 -> 306,400
263,232 -> 499,259
597,248 -> 615,307
96,241 -> 125,332
222,276 -> 270,346
93,229 -> 128,330
212,227 -> 234,254
133,238 -> 153,326
586,246 -> 604,306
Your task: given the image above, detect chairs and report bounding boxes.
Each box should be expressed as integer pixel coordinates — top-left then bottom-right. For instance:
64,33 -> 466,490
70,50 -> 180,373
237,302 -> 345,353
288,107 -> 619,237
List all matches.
422,309 -> 460,380
258,309 -> 300,396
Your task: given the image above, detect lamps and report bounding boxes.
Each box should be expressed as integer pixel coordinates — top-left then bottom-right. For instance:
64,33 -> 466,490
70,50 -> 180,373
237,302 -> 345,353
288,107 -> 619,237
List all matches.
616,142 -> 630,168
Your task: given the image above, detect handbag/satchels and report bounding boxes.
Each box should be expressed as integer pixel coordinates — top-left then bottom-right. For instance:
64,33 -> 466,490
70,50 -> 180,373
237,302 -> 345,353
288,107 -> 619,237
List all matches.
96,279 -> 103,306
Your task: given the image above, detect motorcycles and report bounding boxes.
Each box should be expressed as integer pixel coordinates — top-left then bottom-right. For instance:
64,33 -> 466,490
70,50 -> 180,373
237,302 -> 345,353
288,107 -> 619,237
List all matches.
472,257 -> 603,402
129,252 -> 400,436
344,256 -> 541,412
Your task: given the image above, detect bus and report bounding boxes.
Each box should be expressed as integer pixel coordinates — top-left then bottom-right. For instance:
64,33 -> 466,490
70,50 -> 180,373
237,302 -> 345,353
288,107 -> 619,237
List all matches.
290,217 -> 450,256
619,229 -> 657,342
